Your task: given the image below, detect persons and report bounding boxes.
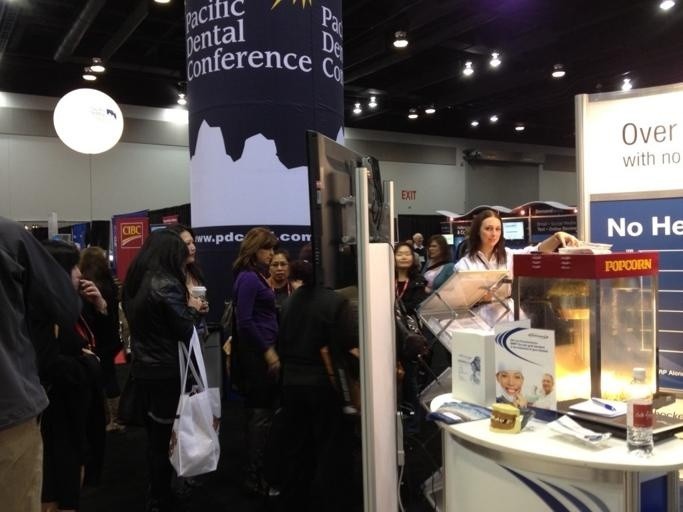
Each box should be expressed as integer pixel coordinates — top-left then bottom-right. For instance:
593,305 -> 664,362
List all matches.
1,221 -> 125,512
532,374 -> 557,409
120,225 -> 216,500
220,228 -> 358,510
496,360 -> 528,409
395,226 -> 472,456
453,210 -> 580,275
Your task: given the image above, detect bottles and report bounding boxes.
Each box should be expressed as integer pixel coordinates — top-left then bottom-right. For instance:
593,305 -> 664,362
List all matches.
624,367 -> 654,459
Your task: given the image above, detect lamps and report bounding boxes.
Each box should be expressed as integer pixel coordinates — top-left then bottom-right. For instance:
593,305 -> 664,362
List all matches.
53,87 -> 124,245
82,66 -> 97,80
89,56 -> 106,72
353,31 -> 633,131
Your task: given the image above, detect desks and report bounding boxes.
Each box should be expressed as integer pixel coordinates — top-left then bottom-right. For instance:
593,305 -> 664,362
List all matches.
430,392 -> 683,512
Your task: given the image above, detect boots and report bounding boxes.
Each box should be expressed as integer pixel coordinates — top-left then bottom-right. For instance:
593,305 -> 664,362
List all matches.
104,395 -> 126,435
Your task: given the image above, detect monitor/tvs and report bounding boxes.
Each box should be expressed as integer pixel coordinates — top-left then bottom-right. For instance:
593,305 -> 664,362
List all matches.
441,234 -> 455,245
305,127 -> 382,291
500,219 -> 526,243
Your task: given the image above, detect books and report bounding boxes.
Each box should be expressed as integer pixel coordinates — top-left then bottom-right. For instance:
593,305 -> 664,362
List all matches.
569,398 -> 627,417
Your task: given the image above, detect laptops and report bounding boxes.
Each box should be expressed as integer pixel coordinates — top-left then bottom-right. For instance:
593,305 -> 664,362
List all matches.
527,398 -> 683,443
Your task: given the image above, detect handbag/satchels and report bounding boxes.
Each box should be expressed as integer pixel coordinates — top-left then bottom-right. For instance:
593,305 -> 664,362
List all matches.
168,387 -> 221,477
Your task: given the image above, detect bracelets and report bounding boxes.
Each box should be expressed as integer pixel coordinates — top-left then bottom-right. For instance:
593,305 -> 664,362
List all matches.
553,232 -> 559,240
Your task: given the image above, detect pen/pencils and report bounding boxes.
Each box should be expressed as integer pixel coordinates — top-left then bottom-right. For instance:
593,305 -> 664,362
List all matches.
592,399 -> 616,412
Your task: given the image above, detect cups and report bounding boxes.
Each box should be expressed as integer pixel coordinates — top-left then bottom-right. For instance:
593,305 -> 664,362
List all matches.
191,285 -> 207,302
488,402 -> 537,435
